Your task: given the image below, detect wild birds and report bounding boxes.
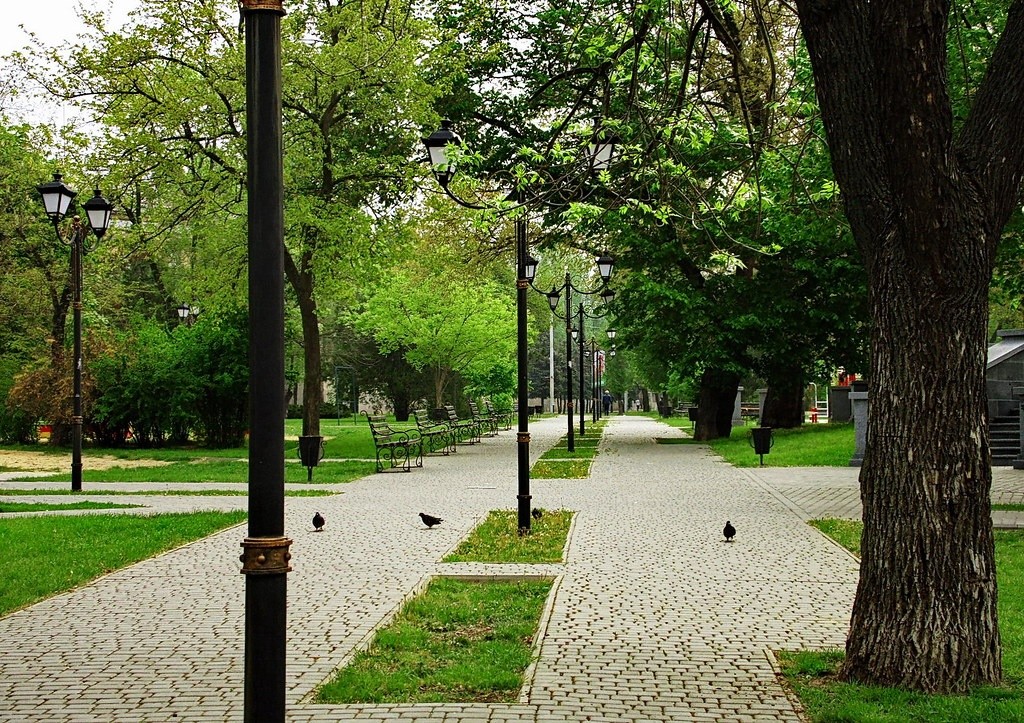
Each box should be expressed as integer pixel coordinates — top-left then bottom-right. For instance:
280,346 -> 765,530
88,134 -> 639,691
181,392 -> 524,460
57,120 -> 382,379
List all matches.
532,507 -> 542,522
311,512 -> 326,532
723,520 -> 736,541
419,512 -> 444,529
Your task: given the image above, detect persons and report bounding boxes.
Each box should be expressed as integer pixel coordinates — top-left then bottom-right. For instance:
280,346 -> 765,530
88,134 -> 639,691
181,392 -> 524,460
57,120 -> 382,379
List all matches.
636,398 -> 643,411
602,390 -> 612,416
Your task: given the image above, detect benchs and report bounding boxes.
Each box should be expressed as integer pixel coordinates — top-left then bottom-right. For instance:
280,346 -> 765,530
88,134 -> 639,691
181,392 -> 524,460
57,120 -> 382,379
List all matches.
410,405 -> 456,456
464,399 -> 498,437
485,401 -> 512,430
360,409 -> 423,473
440,402 -> 481,446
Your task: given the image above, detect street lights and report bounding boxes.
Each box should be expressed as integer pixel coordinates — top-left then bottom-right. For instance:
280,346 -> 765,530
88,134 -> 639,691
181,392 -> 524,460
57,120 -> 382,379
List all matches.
521,248 -> 617,452
35,168 -> 112,494
418,117 -> 629,540
546,284 -> 615,439
569,324 -> 617,425
